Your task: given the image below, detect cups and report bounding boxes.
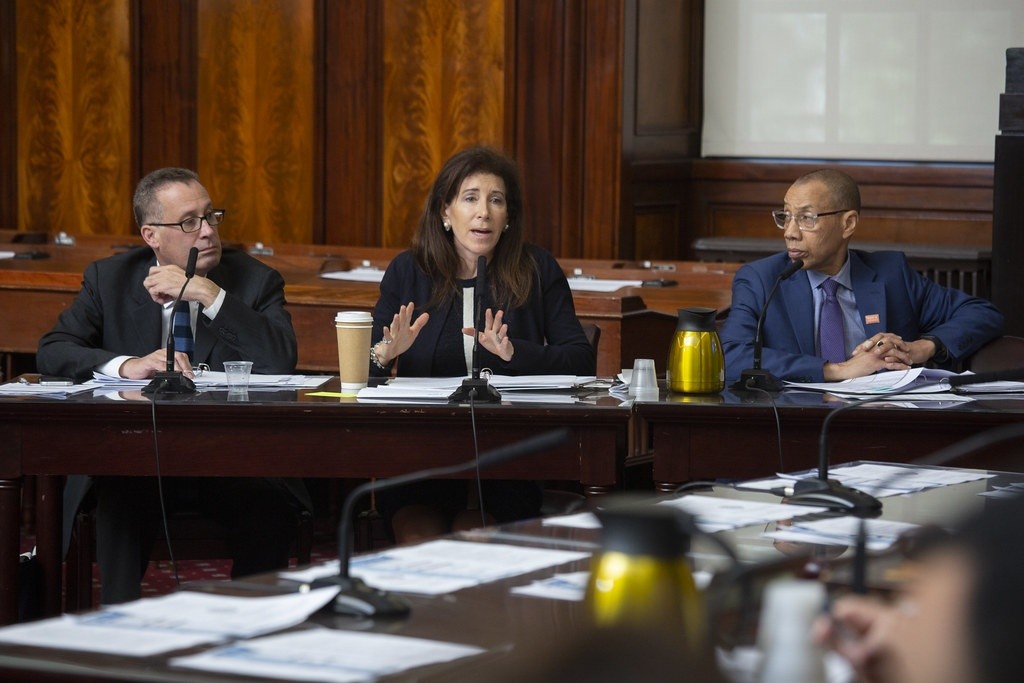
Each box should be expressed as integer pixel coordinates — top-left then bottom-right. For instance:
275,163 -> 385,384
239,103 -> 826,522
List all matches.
335,310 -> 374,390
629,359 -> 659,402
222,361 -> 253,392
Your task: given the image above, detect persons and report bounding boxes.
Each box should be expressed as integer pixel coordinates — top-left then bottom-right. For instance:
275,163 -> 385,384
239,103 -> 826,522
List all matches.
37,166 -> 317,609
720,168 -> 1004,392
811,495 -> 1024,683
369,147 -> 597,546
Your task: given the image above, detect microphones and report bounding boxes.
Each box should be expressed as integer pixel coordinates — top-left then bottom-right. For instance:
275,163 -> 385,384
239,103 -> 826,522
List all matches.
733,258 -> 804,390
780,367 -> 1024,512
447,255 -> 502,402
141,247 -> 199,393
307,430 -> 569,618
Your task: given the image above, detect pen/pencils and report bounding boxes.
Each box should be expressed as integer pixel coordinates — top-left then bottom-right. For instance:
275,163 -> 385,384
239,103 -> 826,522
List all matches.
854,519 -> 867,593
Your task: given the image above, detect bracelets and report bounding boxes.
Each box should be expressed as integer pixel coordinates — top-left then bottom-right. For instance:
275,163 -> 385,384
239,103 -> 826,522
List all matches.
370,347 -> 393,370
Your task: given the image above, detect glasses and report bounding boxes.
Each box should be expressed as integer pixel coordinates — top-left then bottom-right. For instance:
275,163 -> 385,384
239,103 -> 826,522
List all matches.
772,209 -> 853,230
147,208 -> 226,233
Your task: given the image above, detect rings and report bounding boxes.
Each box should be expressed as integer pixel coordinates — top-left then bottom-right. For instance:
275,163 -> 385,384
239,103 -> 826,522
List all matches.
877,342 -> 883,346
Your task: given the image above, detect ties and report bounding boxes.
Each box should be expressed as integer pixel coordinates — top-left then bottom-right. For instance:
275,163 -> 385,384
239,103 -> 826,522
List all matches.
173,300 -> 194,364
819,279 -> 846,364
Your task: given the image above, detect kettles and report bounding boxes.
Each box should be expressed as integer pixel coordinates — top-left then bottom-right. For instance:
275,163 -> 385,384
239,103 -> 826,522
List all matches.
667,306 -> 725,394
585,495 -> 747,671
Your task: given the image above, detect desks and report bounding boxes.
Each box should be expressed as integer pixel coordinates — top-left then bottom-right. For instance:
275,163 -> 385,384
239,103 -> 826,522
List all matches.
0,226 -> 1024,683
